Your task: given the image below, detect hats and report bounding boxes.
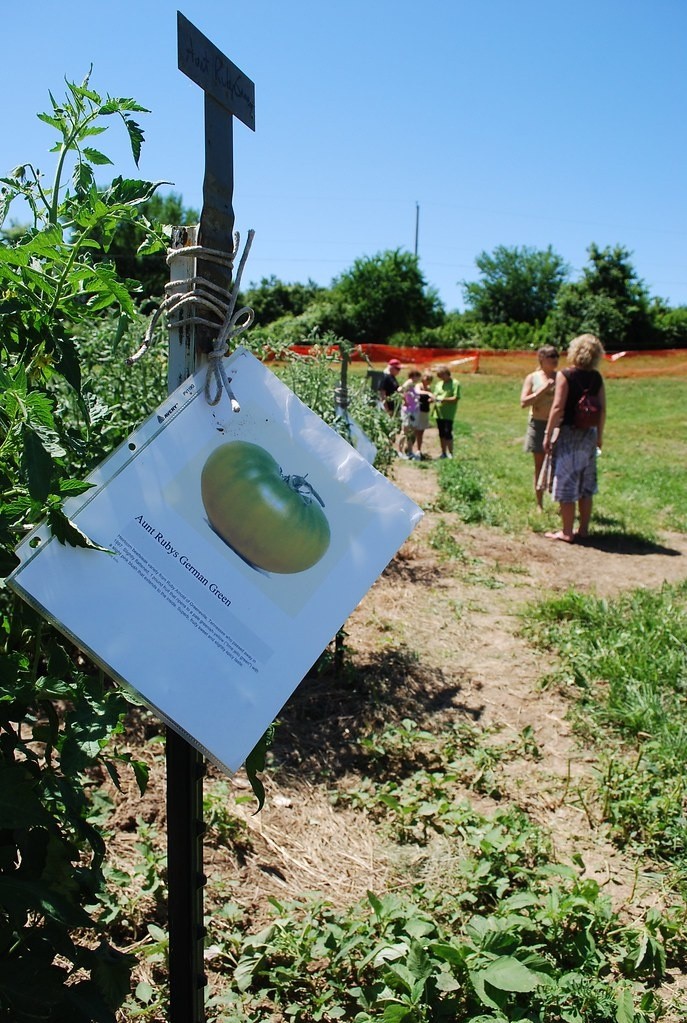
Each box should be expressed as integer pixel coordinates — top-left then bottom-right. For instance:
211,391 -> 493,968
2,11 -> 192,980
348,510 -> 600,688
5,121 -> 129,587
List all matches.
388,359 -> 402,369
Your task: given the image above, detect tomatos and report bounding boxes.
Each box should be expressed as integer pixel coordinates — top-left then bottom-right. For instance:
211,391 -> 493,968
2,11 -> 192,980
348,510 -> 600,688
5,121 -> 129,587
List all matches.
200,440 -> 331,574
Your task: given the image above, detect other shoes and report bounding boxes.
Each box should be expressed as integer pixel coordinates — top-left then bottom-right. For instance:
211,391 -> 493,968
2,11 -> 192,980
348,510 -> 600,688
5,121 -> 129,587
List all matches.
405,448 -> 416,461
416,451 -> 425,461
439,453 -> 446,460
399,453 -> 409,460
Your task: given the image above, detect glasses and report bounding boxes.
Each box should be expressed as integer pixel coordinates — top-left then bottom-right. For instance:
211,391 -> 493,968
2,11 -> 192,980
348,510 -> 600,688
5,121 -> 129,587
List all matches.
394,367 -> 400,371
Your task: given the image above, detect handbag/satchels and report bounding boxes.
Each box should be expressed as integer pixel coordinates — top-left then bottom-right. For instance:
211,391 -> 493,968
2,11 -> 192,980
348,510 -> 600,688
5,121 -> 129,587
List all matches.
536,427 -> 561,494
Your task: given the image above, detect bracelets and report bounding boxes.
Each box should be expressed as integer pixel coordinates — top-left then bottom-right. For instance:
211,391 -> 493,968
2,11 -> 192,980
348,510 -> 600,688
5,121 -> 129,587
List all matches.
545,430 -> 553,436
534,393 -> 541,400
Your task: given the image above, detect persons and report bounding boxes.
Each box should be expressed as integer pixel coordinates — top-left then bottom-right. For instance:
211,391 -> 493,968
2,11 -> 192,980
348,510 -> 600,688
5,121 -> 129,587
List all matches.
521,346 -> 560,515
379,359 -> 462,460
543,334 -> 606,542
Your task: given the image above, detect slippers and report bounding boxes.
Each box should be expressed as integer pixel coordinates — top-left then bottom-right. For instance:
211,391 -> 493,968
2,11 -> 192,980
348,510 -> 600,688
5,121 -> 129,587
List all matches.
573,532 -> 590,541
544,528 -> 574,542
